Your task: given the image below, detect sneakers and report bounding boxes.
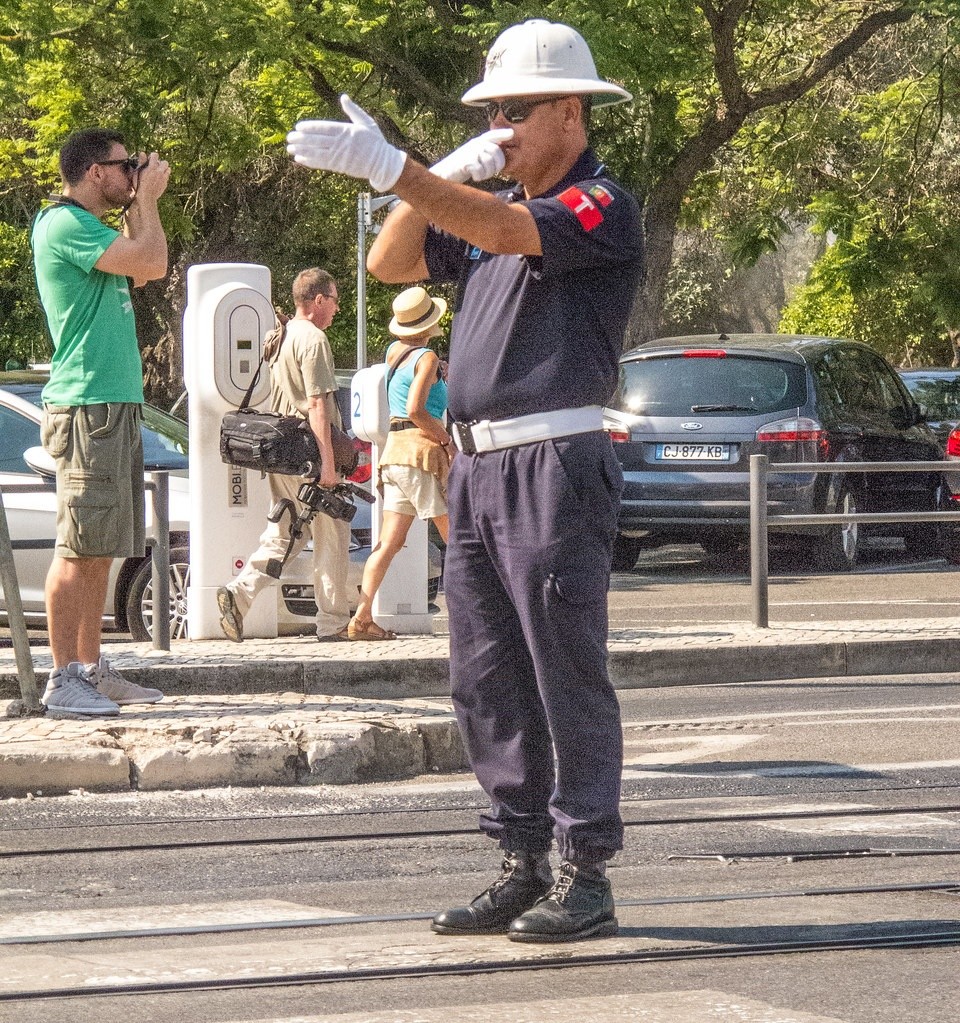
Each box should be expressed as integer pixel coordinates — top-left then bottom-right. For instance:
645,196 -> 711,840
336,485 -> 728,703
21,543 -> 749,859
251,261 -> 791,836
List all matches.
317,626 -> 348,642
217,587 -> 244,644
85,657 -> 164,705
42,662 -> 120,715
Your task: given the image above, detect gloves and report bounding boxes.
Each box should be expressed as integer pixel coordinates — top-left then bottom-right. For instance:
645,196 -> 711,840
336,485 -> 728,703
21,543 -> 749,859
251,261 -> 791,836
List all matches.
427,128 -> 514,185
285,94 -> 408,194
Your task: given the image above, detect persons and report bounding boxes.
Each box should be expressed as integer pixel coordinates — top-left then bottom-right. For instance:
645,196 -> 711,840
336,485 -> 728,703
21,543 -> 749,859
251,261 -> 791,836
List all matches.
30,129 -> 195,715
219,269 -> 355,641
348,286 -> 455,640
284,18 -> 647,944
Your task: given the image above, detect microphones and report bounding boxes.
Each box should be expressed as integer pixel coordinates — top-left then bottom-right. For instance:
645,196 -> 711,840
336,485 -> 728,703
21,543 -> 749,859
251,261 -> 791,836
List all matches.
347,484 -> 376,504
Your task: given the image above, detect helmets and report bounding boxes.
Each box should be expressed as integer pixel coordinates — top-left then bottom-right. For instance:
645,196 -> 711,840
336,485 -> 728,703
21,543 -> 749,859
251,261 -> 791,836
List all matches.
460,18 -> 633,109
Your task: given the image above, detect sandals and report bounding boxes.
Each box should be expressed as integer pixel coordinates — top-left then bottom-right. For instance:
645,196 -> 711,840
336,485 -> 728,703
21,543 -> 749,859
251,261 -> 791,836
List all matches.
347,617 -> 398,641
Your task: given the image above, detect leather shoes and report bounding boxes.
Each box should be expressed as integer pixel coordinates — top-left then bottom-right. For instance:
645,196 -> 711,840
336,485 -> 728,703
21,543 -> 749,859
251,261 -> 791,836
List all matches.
507,859 -> 618,941
431,848 -> 556,935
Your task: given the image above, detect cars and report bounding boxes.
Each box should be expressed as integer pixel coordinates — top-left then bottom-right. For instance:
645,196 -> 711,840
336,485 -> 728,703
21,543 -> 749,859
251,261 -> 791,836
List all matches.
888,367 -> 960,499
599,331 -> 960,574
0,384 -> 443,643
328,368 -> 391,547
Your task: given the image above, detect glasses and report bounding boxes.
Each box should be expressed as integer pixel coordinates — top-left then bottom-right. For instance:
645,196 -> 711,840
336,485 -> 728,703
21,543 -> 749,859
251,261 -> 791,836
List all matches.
324,292 -> 341,307
484,93 -> 575,123
86,156 -> 134,173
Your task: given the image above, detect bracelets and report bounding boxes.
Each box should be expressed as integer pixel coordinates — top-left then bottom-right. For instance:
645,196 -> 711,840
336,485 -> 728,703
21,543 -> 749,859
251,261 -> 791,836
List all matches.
440,435 -> 452,446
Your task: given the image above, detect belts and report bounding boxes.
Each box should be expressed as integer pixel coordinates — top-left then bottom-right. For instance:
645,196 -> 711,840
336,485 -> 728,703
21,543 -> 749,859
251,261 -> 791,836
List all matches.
452,403 -> 603,456
389,421 -> 416,430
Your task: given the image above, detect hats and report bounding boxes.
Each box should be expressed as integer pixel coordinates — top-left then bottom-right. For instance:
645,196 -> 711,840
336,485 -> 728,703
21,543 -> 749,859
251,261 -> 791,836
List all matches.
264,313 -> 289,367
389,287 -> 448,338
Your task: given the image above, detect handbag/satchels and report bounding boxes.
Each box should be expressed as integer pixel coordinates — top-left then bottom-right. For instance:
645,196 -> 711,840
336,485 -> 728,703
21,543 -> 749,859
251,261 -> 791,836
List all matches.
220,409 -> 320,479
330,422 -> 360,477
